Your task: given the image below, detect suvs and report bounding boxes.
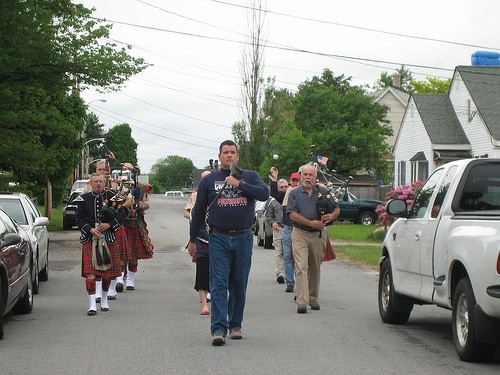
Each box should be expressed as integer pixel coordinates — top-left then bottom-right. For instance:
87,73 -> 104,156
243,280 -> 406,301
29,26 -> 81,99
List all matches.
327,186 -> 385,226
0,191 -> 50,294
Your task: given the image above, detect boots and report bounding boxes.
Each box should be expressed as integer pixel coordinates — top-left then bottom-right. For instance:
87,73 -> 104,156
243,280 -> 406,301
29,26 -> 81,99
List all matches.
107,279 -> 117,300
123,267 -> 127,279
96,281 -> 102,302
116,276 -> 123,292
87,294 -> 97,315
101,291 -> 109,311
125,271 -> 136,289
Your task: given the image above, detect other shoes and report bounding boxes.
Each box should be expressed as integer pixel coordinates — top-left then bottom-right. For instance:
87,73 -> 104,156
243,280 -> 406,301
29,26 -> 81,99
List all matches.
229,328 -> 243,339
309,303 -> 321,310
294,296 -> 296,301
286,285 -> 294,292
297,306 -> 308,313
206,292 -> 210,300
212,332 -> 226,346
199,308 -> 209,315
276,276 -> 285,283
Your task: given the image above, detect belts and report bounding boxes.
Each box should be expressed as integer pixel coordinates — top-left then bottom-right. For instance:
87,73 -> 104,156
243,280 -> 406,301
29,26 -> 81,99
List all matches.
293,225 -> 321,232
208,226 -> 252,236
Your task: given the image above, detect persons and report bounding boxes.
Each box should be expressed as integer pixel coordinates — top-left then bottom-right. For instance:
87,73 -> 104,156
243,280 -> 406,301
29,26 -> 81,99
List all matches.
286,165 -> 340,313
184,170 -> 212,315
188,140 -> 269,347
270,167 -> 299,292
264,179 -> 289,284
82,161 -> 153,303
73,174 -> 130,315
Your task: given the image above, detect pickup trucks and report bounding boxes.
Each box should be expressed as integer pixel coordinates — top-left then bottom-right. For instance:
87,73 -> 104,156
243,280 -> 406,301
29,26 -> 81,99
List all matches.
377,158 -> 500,363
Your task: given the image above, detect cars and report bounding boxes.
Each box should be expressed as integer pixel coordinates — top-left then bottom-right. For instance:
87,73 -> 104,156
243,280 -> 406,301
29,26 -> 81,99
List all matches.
62,191 -> 89,230
0,209 -> 34,340
253,197 -> 274,249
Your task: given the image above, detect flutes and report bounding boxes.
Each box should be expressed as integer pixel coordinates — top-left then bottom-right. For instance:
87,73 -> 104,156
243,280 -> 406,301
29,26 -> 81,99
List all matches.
310,144 -> 354,238
97,163 -> 140,224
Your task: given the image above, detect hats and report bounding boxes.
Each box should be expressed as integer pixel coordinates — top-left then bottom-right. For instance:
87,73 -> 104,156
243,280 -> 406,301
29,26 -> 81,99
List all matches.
297,165 -> 304,173
290,173 -> 299,180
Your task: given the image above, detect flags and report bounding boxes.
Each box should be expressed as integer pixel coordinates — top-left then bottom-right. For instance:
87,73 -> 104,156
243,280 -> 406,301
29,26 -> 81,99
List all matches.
316,155 -> 328,165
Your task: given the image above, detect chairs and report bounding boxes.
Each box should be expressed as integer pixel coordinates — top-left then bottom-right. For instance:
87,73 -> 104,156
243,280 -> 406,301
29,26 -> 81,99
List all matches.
460,182 -> 483,209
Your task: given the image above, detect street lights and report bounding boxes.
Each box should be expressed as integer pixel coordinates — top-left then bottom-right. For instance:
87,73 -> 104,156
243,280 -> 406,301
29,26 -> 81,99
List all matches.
81,137 -> 105,180
75,99 -> 107,187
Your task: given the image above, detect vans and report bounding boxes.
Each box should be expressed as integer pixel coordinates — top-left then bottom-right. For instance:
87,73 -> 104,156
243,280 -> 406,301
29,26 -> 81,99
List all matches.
165,190 -> 183,197
69,179 -> 89,195
